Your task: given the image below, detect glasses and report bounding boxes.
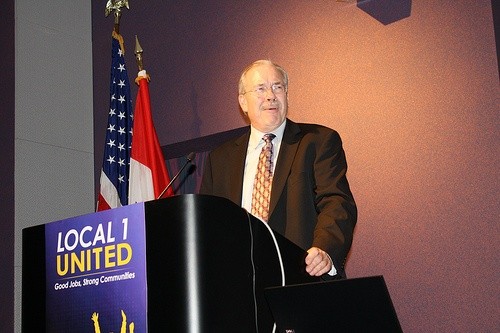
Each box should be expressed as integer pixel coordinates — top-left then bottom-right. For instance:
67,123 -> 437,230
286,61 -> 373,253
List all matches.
242,83 -> 288,94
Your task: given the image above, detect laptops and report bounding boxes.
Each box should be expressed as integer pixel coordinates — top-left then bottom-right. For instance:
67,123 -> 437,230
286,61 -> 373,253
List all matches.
264,274 -> 404,333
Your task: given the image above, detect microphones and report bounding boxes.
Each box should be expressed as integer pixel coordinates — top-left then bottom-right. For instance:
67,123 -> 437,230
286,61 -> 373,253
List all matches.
157,151 -> 195,199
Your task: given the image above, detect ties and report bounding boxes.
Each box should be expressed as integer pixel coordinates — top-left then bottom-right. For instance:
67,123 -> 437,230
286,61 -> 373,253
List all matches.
251,132 -> 276,224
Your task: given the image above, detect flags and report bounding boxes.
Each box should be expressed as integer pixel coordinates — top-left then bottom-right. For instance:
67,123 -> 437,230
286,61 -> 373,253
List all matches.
96,0 -> 177,213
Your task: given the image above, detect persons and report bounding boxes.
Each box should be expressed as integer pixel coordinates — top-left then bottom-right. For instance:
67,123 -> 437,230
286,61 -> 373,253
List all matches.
199,60 -> 357,283
